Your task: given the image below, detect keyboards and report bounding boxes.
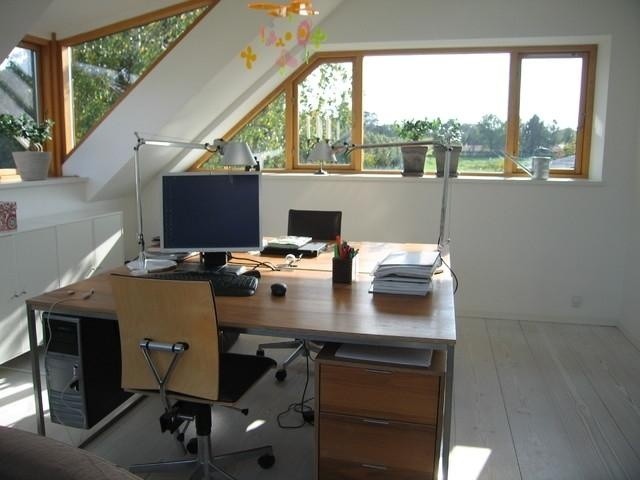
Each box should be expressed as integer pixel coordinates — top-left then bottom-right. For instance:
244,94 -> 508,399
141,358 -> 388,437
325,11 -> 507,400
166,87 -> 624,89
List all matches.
145,271 -> 258,296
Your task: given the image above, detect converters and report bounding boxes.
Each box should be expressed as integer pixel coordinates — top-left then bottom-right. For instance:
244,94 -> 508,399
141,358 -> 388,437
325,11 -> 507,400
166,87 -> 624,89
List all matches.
301,410 -> 315,423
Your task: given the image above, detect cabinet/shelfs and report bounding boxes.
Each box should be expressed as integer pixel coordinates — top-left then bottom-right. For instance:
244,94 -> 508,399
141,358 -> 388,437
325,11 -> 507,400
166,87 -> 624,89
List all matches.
58,208 -> 125,290
313,341 -> 446,480
0,218 -> 58,368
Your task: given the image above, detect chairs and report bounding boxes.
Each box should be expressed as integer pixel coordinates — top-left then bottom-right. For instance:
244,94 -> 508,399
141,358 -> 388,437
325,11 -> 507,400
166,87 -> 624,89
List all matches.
286,207 -> 342,238
112,267 -> 279,480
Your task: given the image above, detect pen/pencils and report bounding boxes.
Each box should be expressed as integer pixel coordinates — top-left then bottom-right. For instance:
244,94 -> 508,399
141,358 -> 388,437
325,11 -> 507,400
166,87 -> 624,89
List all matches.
333,236 -> 359,259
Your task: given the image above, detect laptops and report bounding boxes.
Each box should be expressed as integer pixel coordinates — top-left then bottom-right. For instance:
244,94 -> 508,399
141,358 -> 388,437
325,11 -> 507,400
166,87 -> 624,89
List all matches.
261,241 -> 327,257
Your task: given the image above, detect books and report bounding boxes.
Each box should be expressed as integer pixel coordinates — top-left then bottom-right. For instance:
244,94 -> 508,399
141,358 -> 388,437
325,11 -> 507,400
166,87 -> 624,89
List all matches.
367,248 -> 442,296
268,235 -> 312,249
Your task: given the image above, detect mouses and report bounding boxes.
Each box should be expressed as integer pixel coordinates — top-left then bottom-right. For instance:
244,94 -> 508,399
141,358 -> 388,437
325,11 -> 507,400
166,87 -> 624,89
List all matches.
271,283 -> 287,296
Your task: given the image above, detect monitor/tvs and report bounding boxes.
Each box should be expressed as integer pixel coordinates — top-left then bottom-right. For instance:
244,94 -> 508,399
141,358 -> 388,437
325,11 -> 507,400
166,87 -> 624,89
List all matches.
160,171 -> 264,276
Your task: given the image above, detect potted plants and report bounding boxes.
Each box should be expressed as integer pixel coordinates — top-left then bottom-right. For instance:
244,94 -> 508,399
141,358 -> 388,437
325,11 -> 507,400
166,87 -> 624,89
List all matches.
0,110 -> 58,183
395,118 -> 433,177
430,119 -> 466,176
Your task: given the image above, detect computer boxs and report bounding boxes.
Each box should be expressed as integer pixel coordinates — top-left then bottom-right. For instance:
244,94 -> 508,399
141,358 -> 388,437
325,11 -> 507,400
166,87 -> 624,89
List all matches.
42,313 -> 135,430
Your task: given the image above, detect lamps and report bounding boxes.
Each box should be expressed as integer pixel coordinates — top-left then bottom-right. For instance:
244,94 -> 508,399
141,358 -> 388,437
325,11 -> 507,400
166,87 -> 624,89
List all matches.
127,129 -> 256,268
309,133 -> 452,252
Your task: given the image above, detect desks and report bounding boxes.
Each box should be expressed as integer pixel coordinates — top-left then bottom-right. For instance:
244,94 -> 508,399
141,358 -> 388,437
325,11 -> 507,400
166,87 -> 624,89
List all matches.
23,268 -> 455,479
226,239 -> 454,269
